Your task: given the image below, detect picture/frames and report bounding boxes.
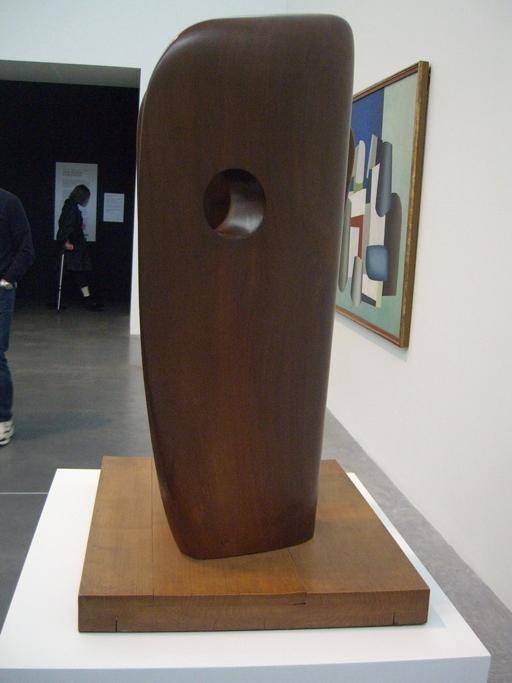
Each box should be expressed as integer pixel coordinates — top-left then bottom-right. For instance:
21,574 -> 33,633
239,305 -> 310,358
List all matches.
336,59 -> 429,352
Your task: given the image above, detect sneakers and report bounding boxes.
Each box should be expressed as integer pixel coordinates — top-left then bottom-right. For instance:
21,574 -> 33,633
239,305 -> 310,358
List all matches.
0,421 -> 17,444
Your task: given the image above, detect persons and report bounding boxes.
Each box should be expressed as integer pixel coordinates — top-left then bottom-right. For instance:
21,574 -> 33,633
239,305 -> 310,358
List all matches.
0,189 -> 33,445
57,186 -> 104,311
